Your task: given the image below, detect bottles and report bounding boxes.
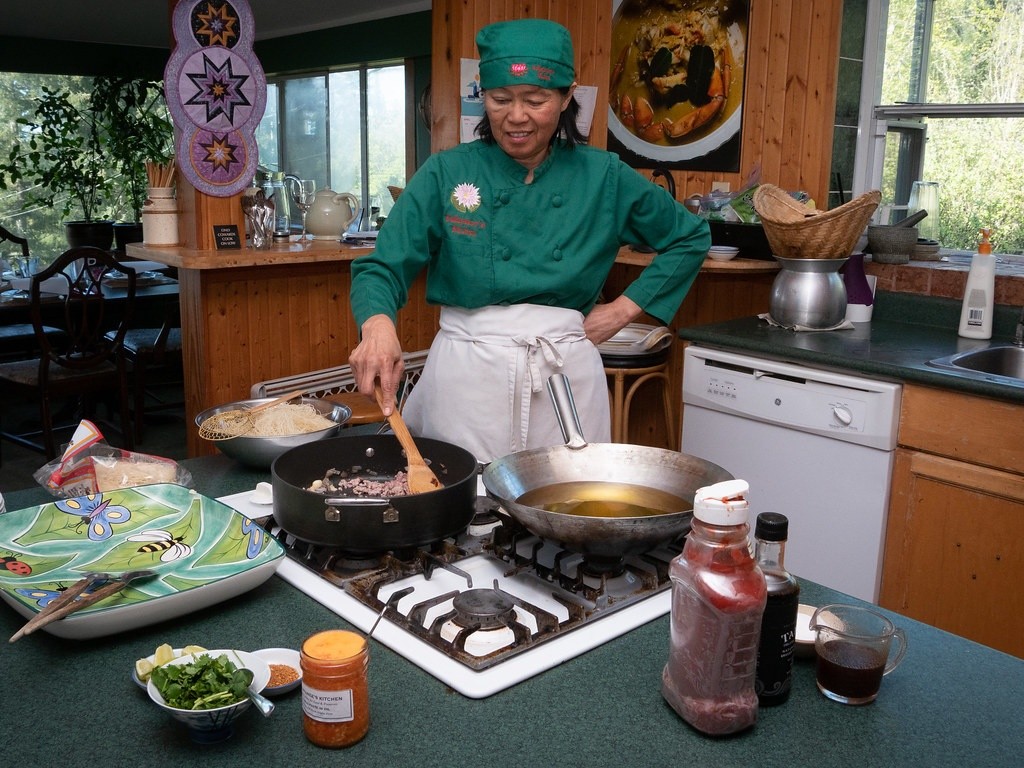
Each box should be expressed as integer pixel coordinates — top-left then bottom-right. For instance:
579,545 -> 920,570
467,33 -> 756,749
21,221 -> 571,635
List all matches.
684,199 -> 700,216
769,254 -> 873,329
142,187 -> 179,248
660,479 -> 800,733
301,629 -> 369,747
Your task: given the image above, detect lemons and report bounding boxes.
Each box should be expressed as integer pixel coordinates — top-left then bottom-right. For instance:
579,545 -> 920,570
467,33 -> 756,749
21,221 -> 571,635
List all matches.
135,658 -> 155,681
152,643 -> 174,666
180,645 -> 207,655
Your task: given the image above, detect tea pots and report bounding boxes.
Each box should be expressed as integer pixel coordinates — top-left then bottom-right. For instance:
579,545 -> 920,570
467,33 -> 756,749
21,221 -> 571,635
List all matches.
305,186 -> 359,240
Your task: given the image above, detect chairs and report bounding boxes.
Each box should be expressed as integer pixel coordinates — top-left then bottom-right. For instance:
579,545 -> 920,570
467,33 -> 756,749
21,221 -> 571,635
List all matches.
0,225 -> 186,465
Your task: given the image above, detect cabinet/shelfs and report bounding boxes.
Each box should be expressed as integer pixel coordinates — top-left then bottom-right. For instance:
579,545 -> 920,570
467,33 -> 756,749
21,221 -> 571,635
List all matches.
880,382 -> 1024,656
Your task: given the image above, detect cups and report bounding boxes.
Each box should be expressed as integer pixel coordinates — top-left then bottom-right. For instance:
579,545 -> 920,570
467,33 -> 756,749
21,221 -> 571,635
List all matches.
907,182 -> 940,242
809,604 -> 908,705
70,257 -> 85,283
249,207 -> 275,249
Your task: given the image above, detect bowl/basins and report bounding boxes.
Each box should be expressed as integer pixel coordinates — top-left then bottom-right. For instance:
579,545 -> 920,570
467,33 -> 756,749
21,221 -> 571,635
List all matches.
851,226 -> 868,255
708,246 -> 739,262
132,649 -> 182,691
251,648 -> 303,696
195,397 -> 352,469
795,604 -> 846,655
148,649 -> 271,729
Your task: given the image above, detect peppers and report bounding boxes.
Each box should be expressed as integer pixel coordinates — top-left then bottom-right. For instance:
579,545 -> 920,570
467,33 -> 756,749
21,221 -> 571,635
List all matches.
708,186 -> 764,222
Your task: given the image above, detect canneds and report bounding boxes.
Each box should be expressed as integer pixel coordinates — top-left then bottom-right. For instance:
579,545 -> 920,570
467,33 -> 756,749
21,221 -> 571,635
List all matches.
300,628 -> 369,749
683,199 -> 701,215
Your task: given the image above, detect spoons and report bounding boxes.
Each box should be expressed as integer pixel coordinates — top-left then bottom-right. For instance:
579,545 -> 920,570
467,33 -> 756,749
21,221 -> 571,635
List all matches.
9,570 -> 158,644
233,668 -> 275,717
242,190 -> 275,249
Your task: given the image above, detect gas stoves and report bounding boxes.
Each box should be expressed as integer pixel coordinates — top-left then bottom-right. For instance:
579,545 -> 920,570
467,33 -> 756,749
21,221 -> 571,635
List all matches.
214,461 -> 690,699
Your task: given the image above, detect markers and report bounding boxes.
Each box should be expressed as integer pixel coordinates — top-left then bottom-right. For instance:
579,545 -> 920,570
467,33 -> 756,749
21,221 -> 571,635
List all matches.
346,236 -> 376,241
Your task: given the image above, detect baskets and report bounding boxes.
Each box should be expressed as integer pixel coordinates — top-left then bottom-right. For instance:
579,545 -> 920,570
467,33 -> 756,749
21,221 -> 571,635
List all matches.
753,182 -> 882,259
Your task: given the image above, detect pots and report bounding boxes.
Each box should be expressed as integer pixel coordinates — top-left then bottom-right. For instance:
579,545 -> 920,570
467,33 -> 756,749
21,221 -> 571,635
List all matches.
271,374 -> 736,549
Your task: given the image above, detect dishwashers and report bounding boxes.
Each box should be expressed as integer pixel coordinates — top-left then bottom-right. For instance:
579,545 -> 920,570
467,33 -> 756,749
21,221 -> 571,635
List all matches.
681,345 -> 902,606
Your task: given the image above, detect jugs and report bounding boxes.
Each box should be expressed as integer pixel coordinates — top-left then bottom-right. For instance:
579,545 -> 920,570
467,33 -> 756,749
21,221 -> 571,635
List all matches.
256,164 -> 304,241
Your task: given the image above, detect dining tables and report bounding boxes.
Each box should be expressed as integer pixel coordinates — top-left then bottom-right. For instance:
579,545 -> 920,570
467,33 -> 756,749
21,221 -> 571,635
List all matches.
0,275 -> 179,357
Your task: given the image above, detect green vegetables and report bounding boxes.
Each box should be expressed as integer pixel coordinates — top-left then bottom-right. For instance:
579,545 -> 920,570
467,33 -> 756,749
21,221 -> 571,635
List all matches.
150,649 -> 251,710
644,44 -> 715,106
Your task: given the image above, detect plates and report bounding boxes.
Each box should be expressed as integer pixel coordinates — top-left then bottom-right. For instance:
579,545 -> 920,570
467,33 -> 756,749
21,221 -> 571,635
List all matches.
608,0 -> 742,161
1,483 -> 287,639
106,271 -> 163,283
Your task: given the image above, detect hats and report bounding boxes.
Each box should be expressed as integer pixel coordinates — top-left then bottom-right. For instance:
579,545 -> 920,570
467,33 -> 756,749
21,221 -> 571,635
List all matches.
476,19 -> 575,90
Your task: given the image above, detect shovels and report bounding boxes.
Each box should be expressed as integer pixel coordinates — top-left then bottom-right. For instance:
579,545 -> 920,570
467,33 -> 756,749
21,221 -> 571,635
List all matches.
351,349 -> 443,495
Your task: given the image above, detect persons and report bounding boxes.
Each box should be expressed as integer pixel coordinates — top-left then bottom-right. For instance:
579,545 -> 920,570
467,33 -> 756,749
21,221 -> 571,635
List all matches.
348,18 -> 711,473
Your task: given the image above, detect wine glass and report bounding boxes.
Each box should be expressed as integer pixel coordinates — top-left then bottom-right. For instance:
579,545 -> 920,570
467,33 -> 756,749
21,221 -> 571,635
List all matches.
15,257 -> 40,294
290,180 -> 316,244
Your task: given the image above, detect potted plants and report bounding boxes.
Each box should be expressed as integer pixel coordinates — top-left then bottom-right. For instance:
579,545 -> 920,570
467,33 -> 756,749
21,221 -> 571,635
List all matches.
0,69 -> 179,252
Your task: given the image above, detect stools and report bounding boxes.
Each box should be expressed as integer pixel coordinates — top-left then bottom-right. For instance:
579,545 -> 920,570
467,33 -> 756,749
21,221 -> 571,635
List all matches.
600,347 -> 676,450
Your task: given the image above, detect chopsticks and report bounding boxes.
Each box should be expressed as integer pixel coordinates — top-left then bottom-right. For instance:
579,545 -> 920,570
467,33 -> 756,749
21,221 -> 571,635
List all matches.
144,160 -> 178,188
